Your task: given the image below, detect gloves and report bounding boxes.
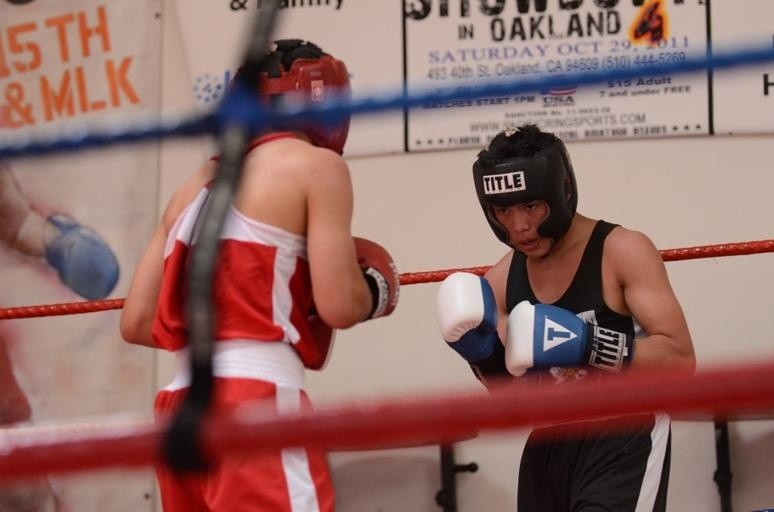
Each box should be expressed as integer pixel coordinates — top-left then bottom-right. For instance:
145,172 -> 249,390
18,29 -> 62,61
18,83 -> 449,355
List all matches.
353,236 -> 401,320
504,300 -> 627,378
436,271 -> 499,363
41,211 -> 118,301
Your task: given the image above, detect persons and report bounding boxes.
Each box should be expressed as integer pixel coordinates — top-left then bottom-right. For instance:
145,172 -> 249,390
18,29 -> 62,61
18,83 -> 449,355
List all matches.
116,36 -> 400,511
437,118 -> 696,512
1,156 -> 123,512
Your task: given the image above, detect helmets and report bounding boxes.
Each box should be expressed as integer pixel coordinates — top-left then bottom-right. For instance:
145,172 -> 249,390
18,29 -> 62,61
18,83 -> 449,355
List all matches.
472,130 -> 578,247
258,55 -> 352,155
